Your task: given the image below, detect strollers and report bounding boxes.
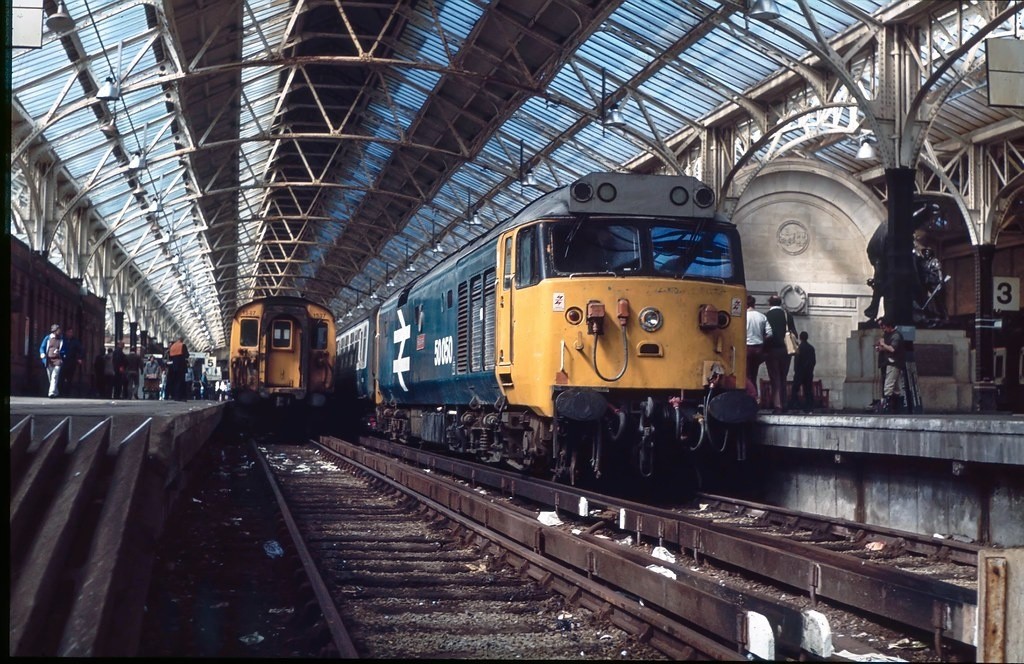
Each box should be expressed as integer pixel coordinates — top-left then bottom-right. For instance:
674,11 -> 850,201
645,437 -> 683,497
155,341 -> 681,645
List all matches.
142,374 -> 162,401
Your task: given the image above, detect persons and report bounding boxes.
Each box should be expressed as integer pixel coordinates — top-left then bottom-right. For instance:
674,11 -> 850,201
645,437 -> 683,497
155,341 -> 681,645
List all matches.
39,324 -> 233,401
742,294 -> 911,413
922,248 -> 946,325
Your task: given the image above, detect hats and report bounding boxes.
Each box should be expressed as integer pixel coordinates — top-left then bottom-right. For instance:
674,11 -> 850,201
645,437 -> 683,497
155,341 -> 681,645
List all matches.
50,324 -> 59,332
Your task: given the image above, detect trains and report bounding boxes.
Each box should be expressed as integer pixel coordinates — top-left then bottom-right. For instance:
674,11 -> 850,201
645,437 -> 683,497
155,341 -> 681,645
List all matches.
337,172 -> 759,492
228,291 -> 338,442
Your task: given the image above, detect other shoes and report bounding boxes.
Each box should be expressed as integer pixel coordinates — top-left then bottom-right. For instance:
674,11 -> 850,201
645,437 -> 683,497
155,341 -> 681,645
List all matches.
772,406 -> 781,415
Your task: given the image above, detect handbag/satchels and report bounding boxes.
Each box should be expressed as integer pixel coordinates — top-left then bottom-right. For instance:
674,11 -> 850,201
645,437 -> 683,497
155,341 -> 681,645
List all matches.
783,331 -> 800,356
